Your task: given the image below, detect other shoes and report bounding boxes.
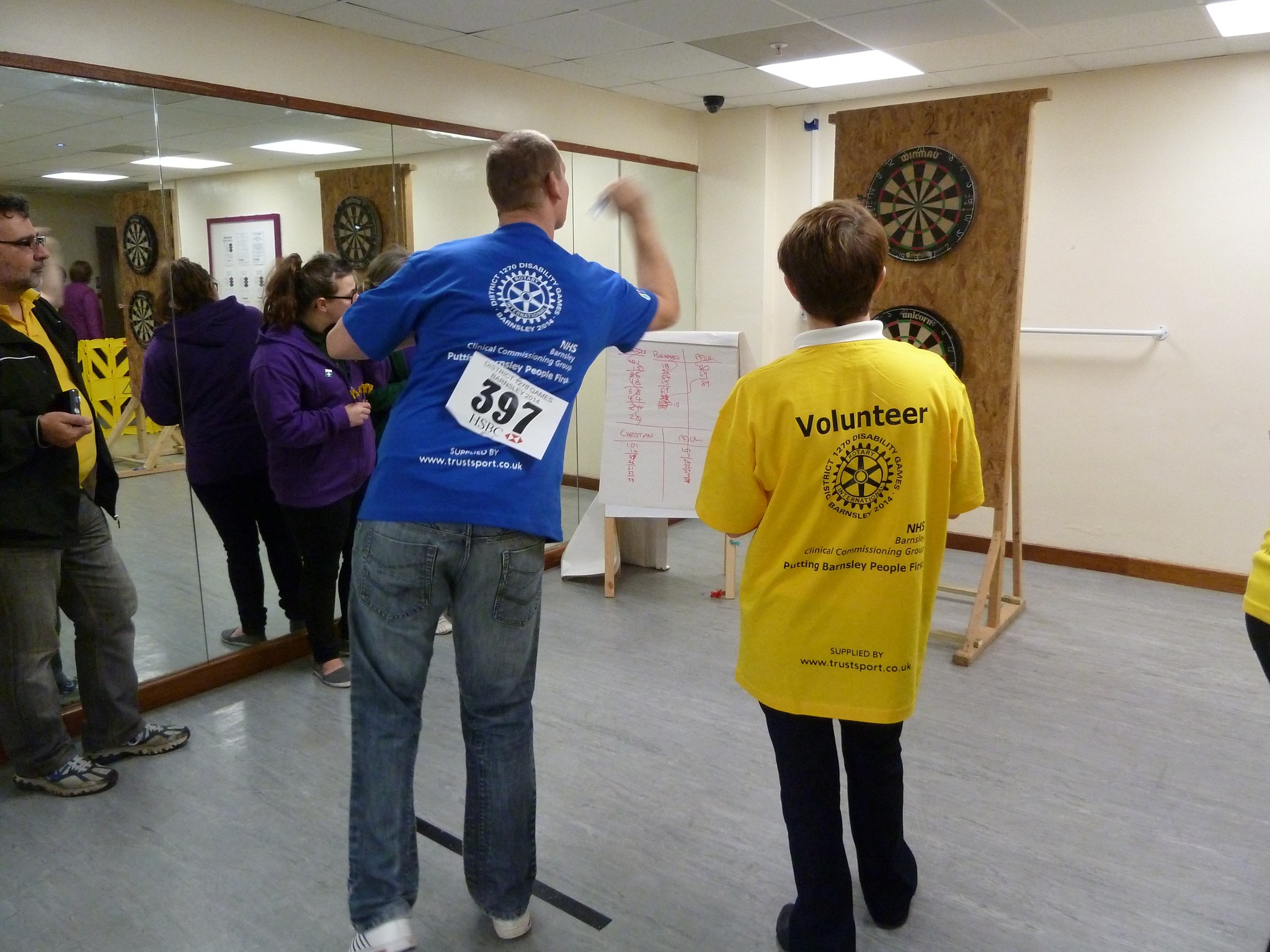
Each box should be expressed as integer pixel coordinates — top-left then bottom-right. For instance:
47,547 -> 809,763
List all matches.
221,626 -> 268,647
435,614 -> 453,635
776,903 -> 795,952
313,657 -> 351,688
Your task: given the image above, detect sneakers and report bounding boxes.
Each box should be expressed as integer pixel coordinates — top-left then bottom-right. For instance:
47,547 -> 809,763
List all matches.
492,909 -> 532,939
14,754 -> 119,797
60,676 -> 78,693
349,917 -> 417,952
87,723 -> 190,766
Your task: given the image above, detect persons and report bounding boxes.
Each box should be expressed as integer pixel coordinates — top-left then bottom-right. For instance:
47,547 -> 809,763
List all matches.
139,256 -> 306,648
247,249 -> 453,687
1243,529 -> 1270,687
50,605 -> 82,706
58,259 -> 106,339
323,127 -> 678,952
0,191 -> 190,797
694,201 -> 987,952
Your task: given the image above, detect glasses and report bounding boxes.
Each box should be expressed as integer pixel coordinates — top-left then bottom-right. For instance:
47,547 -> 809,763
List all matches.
0,235 -> 46,250
213,282 -> 218,291
314,285 -> 358,304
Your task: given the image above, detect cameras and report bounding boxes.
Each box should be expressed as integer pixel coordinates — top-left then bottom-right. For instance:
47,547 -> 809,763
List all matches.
55,389 -> 82,417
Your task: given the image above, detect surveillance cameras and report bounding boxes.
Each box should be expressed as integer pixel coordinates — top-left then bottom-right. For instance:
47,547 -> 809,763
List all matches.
703,95 -> 725,114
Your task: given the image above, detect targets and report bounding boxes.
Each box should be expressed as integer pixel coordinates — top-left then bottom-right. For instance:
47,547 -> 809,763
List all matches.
871,306 -> 963,380
864,145 -> 976,263
331,197 -> 384,269
128,292 -> 156,348
123,214 -> 156,274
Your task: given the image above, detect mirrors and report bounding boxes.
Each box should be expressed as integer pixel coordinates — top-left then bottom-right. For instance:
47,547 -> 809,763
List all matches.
0,52 -> 699,736
